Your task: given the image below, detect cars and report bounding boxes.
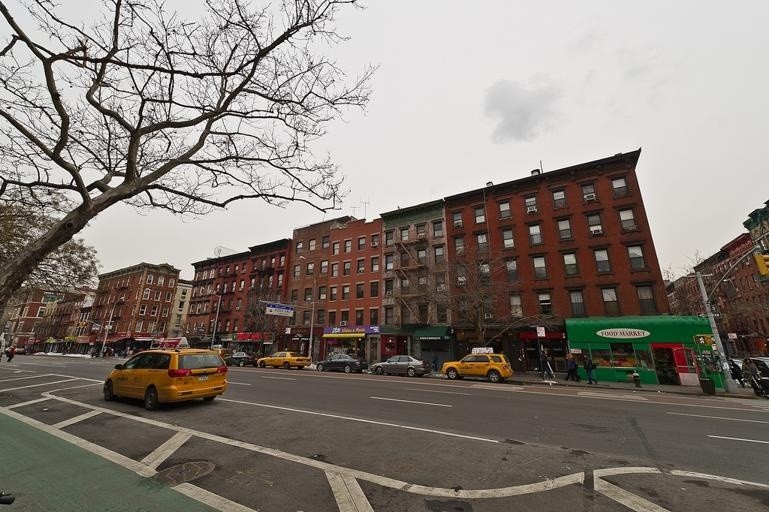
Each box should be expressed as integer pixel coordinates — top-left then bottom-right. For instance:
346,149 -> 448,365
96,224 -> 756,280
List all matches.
224,352 -> 311,369
728,357 -> 768,396
317,354 -> 368,373
103,348 -> 228,410
1,338 -> 26,361
370,355 -> 429,377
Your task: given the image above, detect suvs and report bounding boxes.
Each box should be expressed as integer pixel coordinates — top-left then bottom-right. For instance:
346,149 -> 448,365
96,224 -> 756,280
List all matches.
442,353 -> 513,383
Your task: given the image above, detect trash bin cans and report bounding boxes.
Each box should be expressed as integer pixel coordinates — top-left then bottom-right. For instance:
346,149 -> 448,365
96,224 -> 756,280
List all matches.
700,376 -> 714,394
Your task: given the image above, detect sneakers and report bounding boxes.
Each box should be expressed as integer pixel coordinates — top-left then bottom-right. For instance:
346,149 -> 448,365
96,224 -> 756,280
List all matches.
564,379 -> 568,380
588,380 -> 597,384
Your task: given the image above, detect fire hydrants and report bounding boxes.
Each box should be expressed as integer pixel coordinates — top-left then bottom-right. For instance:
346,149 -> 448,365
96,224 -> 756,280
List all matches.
633,370 -> 641,388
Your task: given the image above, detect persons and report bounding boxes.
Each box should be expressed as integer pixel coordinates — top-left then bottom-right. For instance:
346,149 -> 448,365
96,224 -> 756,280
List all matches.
729,359 -> 743,386
740,359 -> 762,388
562,353 -> 581,382
90,345 -> 127,359
542,354 -> 554,380
583,354 -> 598,385
7,344 -> 17,363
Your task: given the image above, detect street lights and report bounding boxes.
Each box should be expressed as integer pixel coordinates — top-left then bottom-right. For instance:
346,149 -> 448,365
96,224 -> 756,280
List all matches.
201,286 -> 222,347
85,320 -> 107,357
695,245 -> 759,393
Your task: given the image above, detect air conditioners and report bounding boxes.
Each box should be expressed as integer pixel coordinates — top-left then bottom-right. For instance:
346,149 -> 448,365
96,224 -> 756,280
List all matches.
457,276 -> 466,286
593,230 -> 600,235
528,206 -> 536,212
339,321 -> 347,326
371,242 -> 377,246
485,313 -> 493,318
585,195 -> 596,201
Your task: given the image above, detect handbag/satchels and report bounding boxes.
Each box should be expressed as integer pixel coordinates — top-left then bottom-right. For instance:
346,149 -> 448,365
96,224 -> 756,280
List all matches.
591,363 -> 596,369
